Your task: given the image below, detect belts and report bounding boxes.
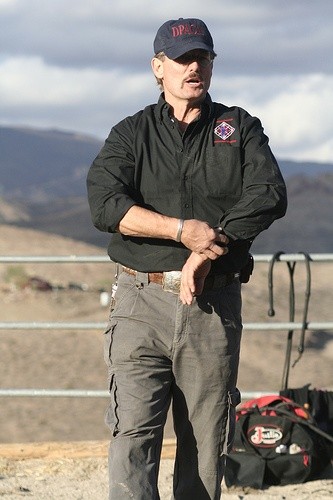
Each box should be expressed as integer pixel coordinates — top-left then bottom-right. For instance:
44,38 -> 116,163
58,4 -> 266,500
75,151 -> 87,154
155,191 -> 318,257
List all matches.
118,265 -> 240,291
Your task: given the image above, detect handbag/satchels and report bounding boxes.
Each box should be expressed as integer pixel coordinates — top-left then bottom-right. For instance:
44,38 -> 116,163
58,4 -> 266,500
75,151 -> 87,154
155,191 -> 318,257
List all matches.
279,384 -> 332,474
224,397 -> 325,489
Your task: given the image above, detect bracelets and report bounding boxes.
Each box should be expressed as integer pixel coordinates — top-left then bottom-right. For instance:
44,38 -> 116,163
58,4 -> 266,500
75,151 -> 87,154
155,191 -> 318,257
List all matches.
175,218 -> 184,242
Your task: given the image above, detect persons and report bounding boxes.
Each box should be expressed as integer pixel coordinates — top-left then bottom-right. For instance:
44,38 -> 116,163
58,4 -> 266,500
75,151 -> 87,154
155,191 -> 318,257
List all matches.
85,19 -> 288,499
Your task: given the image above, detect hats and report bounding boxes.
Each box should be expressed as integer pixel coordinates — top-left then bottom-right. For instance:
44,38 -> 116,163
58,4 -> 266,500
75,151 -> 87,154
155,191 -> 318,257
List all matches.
153,17 -> 214,63
